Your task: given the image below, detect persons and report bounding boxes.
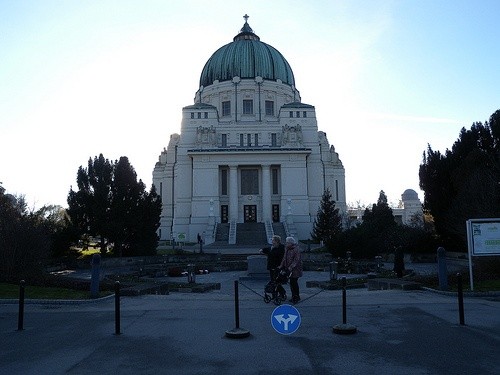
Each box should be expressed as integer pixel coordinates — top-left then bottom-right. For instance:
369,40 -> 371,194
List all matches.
259,235 -> 287,301
277,236 -> 304,303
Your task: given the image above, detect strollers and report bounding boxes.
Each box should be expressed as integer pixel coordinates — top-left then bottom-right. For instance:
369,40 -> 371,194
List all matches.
264,265 -> 288,305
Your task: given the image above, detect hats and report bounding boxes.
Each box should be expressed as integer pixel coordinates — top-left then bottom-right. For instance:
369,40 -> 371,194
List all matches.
285,237 -> 295,244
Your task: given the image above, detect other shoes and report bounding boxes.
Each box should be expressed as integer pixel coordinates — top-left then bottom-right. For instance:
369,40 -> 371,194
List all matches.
278,290 -> 287,296
289,296 -> 301,305
